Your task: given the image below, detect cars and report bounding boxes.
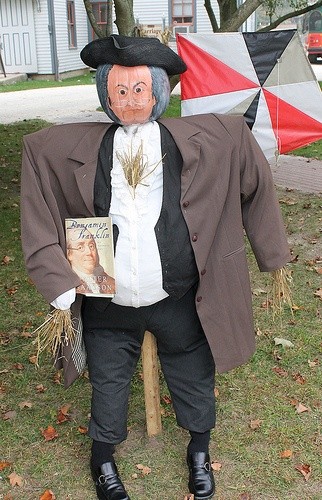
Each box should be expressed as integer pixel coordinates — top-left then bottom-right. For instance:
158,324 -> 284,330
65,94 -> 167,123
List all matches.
308,32 -> 322,64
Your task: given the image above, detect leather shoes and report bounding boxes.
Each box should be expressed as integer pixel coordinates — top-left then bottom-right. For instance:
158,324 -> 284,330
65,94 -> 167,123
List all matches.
89,458 -> 130,500
186,450 -> 215,499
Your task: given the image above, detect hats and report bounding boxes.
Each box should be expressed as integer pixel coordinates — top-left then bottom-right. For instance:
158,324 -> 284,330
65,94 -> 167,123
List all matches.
80,34 -> 187,76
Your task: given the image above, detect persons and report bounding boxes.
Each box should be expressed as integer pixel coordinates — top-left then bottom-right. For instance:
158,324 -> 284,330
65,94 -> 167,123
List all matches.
66,226 -> 115,294
20,32 -> 288,500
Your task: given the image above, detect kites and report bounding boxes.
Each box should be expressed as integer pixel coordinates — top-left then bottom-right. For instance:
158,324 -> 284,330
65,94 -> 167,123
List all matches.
175,32 -> 322,160
